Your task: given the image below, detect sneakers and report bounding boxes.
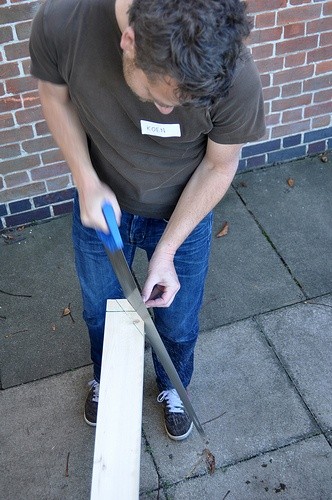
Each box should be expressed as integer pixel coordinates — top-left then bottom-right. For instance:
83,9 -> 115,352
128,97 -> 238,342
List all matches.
158,384 -> 193,441
84,379 -> 101,427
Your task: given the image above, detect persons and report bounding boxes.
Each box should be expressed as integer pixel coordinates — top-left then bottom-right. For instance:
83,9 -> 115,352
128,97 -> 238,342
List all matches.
29,1 -> 265,440
